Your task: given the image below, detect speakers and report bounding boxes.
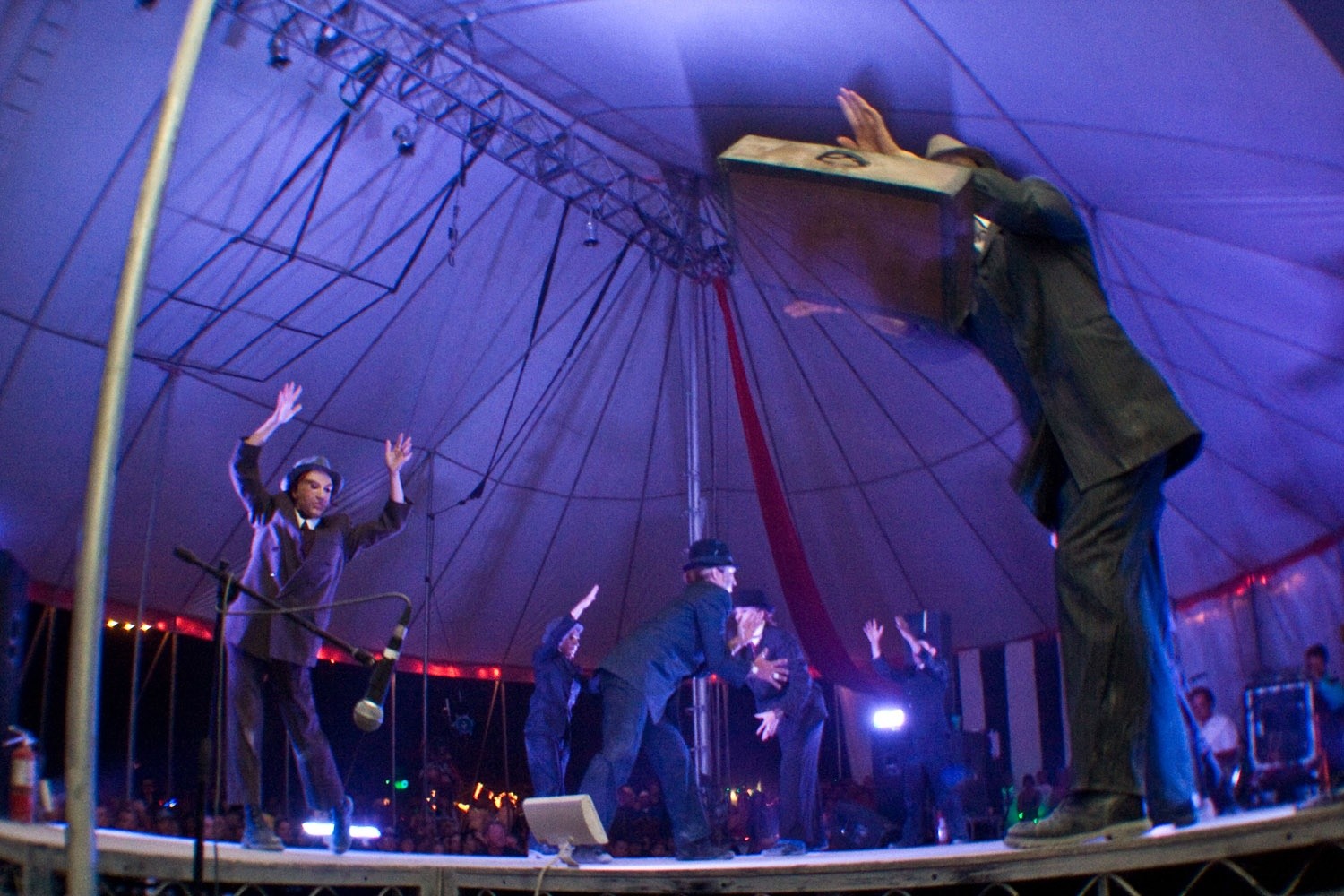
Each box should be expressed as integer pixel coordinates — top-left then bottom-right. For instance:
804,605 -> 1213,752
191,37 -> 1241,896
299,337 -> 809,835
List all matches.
831,803 -> 896,849
958,732 -> 994,818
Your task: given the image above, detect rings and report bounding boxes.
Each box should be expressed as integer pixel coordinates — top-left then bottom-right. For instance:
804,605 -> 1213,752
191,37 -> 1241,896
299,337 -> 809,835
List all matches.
772,671 -> 780,681
761,651 -> 766,658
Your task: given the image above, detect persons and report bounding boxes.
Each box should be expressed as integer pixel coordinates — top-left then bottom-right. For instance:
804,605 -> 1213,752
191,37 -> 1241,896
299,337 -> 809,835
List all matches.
223,381 -> 414,854
38,790 -> 536,895
861,609 -> 968,848
605,784 -> 883,858
733,588 -> 829,856
835,84 -> 1199,848
525,585 -> 599,860
572,538 -> 789,864
964,533 -> 1344,842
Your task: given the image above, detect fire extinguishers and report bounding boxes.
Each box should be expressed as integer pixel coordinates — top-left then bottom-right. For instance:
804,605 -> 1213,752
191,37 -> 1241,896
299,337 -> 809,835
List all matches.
1,726 -> 39,823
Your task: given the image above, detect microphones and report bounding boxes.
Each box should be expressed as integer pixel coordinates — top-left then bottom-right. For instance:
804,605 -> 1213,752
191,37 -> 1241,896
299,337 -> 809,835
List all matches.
355,605 -> 413,733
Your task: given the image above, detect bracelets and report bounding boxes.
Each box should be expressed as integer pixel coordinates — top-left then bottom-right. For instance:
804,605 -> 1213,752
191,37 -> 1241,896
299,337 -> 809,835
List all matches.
770,707 -> 784,720
747,660 -> 758,678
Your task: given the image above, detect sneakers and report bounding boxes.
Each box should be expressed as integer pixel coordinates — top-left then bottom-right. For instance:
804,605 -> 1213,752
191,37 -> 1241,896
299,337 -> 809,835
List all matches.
1004,789 -> 1151,848
1148,797 -> 1198,827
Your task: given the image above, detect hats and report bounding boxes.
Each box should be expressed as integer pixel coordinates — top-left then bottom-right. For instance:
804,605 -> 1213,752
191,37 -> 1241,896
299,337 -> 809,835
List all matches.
282,455 -> 344,500
683,539 -> 742,571
542,617 -> 583,651
733,589 -> 775,611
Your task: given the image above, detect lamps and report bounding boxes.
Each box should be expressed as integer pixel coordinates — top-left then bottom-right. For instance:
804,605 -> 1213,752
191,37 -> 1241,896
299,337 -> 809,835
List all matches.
522,793 -> 610,863
393,113 -> 417,154
265,33 -> 293,72
582,208 -> 602,248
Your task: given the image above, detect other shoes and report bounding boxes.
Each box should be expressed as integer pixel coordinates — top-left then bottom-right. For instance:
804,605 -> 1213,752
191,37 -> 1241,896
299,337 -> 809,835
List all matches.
331,798 -> 353,852
527,841 -> 559,859
571,847 -> 613,864
674,837 -> 734,861
762,843 -> 804,856
889,840 -> 912,848
951,837 -> 967,844
241,826 -> 283,850
808,841 -> 828,851
